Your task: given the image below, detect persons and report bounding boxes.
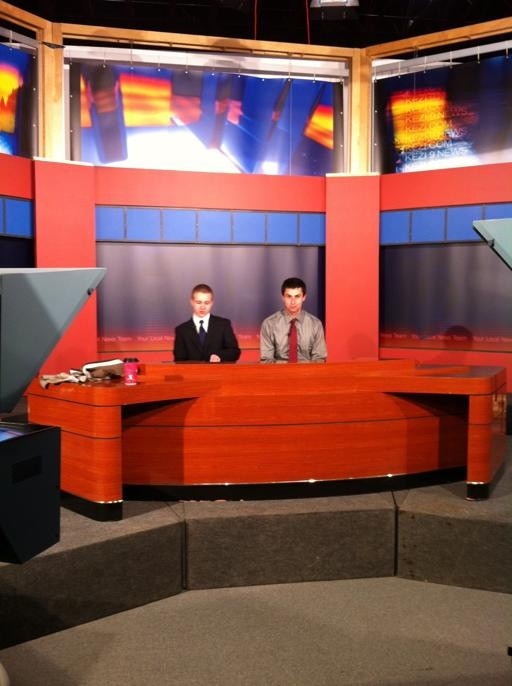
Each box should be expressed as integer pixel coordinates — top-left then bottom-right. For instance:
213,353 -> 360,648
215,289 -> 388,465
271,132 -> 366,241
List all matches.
260,278 -> 327,364
174,284 -> 241,363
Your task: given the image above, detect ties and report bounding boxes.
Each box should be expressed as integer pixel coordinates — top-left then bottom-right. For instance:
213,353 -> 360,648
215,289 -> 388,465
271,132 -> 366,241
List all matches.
288,319 -> 299,364
198,319 -> 207,348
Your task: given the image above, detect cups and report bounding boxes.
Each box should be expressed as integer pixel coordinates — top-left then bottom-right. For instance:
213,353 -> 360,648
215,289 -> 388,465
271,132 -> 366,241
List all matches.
123,358 -> 139,386
